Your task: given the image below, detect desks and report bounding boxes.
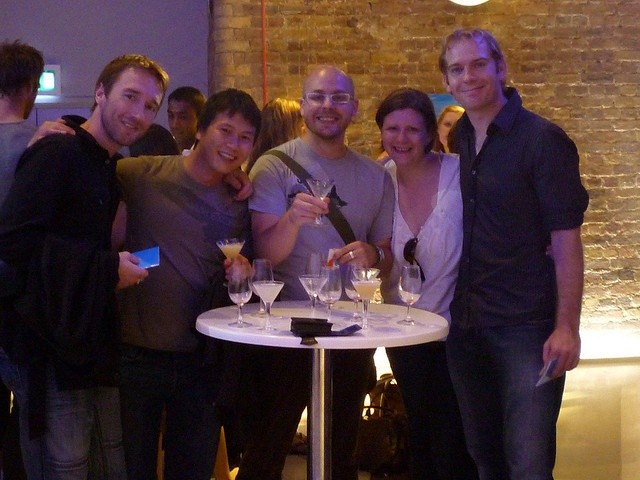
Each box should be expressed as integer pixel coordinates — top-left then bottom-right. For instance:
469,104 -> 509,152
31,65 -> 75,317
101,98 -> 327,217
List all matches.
195,299 -> 450,480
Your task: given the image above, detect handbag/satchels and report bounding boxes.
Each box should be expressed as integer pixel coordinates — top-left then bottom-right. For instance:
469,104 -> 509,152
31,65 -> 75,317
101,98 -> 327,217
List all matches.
349,373 -> 413,473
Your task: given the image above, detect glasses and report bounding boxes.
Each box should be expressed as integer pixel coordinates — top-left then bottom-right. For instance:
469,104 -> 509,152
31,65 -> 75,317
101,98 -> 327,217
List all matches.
304,93 -> 354,105
404,238 -> 425,281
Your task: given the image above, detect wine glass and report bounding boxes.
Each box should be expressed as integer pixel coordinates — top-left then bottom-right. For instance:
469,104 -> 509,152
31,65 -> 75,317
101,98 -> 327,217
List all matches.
344,268 -> 363,321
397,265 -> 423,329
228,267 -> 254,329
252,281 -> 285,333
251,259 -> 274,319
320,267 -> 343,323
298,275 -> 326,316
350,280 -> 380,329
216,236 -> 246,286
352,267 -> 382,281
306,178 -> 338,228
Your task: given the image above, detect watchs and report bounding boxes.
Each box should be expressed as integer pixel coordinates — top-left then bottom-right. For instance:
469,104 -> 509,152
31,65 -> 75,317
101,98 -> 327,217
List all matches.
369,244 -> 384,269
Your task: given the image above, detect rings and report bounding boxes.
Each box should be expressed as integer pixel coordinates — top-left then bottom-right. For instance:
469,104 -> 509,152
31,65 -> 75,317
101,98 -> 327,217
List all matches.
349,250 -> 356,260
136,276 -> 142,285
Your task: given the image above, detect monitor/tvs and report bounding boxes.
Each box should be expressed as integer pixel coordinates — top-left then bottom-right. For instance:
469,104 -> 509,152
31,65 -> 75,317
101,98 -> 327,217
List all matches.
34,64 -> 63,104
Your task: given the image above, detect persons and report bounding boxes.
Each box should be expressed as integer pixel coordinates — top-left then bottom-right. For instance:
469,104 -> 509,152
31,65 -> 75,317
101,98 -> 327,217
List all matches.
375,88 -> 464,479
237,66 -> 396,480
167,87 -> 206,153
244,98 -> 304,175
438,30 -> 588,480
111,89 -> 260,480
435,104 -> 465,154
0,40 -> 43,199
0,54 -> 170,480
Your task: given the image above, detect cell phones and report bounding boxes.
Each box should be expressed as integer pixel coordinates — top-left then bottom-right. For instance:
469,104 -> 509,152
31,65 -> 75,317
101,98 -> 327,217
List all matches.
130,246 -> 160,270
